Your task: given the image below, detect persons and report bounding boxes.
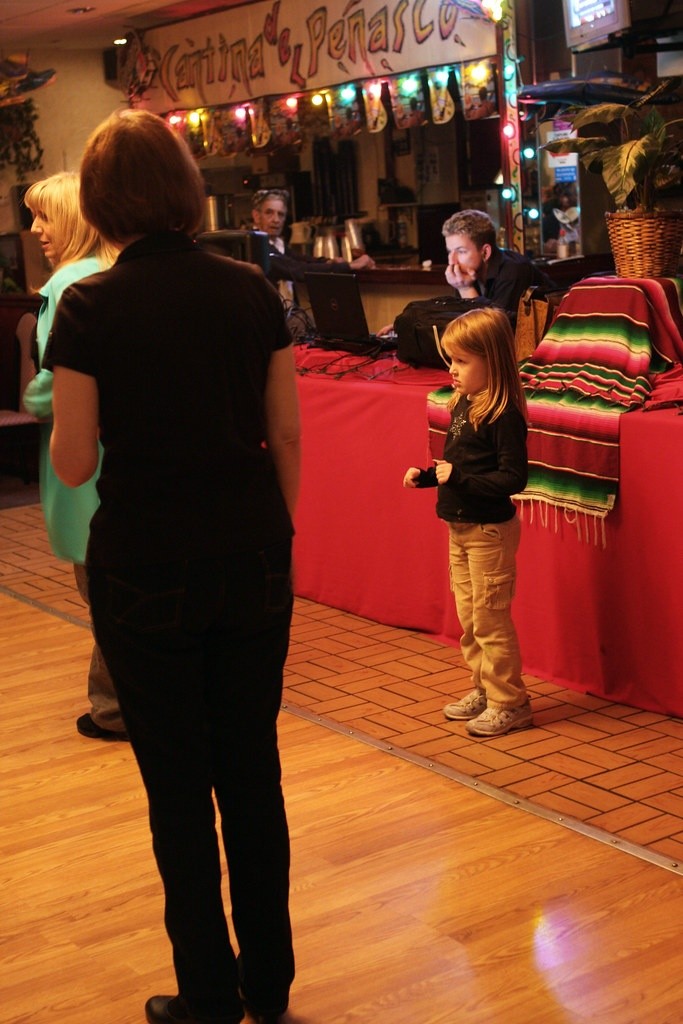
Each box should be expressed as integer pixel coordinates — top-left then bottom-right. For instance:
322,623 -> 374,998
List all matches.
240,191 -> 375,323
403,309 -> 530,736
42,113 -> 298,1024
23,171 -> 123,746
377,209 -> 549,340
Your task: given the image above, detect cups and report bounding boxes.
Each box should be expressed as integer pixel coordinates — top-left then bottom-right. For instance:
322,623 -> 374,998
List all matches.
313,218 -> 364,263
203,191 -> 232,232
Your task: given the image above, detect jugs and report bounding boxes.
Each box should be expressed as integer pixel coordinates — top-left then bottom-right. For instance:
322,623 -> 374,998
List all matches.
287,221 -> 319,261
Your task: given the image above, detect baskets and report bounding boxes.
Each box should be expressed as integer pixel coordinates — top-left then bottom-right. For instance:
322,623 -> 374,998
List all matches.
604,206 -> 683,279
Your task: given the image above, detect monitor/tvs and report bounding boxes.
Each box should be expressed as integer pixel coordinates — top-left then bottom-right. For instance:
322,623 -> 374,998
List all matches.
561,0 -> 631,53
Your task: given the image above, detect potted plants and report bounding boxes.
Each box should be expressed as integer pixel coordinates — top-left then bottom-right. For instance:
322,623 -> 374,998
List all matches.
534,80 -> 683,280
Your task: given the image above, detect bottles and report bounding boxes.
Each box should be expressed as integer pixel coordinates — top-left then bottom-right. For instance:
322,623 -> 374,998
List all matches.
397,216 -> 408,248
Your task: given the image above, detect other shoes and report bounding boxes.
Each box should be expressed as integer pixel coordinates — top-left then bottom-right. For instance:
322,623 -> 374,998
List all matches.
75,711 -> 129,741
145,993 -> 181,1023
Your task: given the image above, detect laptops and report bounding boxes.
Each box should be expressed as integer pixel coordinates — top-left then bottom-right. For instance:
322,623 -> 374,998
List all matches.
303,271 -> 398,346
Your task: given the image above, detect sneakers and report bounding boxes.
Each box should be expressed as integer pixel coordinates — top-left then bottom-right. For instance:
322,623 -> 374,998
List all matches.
442,688 -> 488,720
465,693 -> 535,736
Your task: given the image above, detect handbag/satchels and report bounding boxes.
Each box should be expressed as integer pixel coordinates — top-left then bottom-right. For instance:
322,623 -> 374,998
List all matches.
394,296 -> 519,372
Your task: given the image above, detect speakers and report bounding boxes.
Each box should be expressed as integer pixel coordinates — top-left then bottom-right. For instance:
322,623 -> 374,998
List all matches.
104,47 -> 125,81
